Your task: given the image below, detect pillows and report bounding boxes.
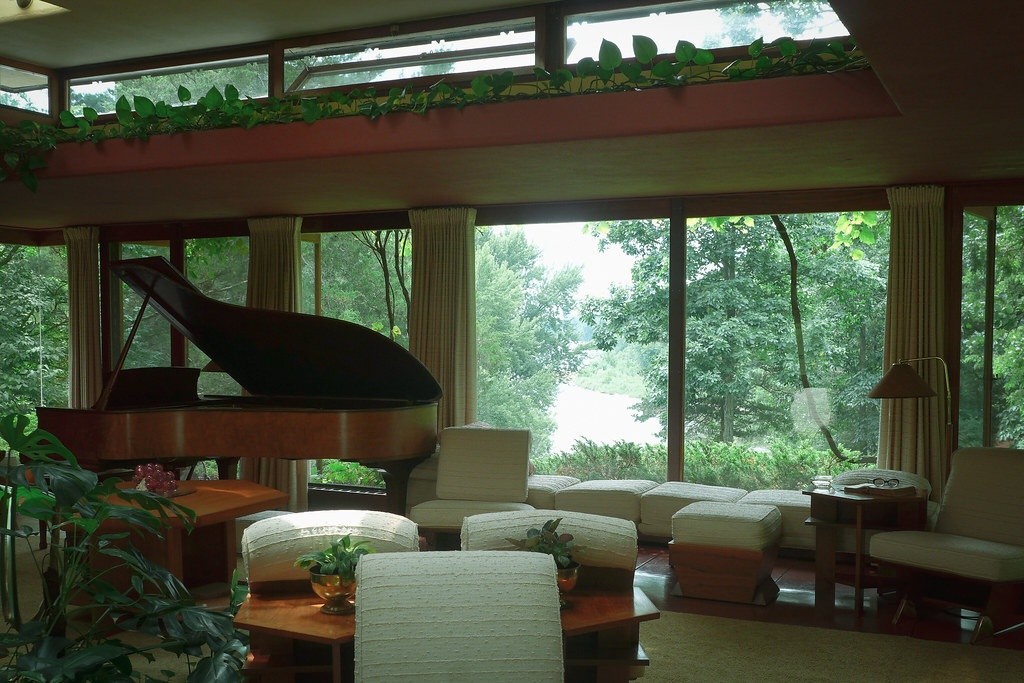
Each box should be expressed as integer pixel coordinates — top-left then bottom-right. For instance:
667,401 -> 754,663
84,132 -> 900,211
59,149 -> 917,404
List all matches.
831,469 -> 933,498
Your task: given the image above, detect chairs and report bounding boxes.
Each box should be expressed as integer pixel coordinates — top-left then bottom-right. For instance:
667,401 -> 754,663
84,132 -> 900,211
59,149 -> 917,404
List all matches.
459,510 -> 638,584
240,509 -> 427,595
409,427 -> 532,550
868,447 -> 1024,645
354,551 -> 565,683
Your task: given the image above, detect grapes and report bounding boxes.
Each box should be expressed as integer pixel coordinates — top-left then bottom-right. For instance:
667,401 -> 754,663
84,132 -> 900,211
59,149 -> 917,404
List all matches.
132,462 -> 179,494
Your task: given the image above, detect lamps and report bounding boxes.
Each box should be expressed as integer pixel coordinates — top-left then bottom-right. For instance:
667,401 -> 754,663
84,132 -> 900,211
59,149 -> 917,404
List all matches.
868,355 -> 952,485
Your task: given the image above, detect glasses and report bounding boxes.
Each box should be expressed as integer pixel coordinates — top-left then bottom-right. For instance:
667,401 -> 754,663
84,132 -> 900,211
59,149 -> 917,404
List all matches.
867,478 -> 899,487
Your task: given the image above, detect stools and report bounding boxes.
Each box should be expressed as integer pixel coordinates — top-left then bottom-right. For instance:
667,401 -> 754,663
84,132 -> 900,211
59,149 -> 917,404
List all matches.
735,490 -> 816,559
406,444 -> 440,519
668,501 -> 782,604
637,481 -> 748,538
835,500 -> 940,564
524,475 -> 581,511
555,480 -> 660,524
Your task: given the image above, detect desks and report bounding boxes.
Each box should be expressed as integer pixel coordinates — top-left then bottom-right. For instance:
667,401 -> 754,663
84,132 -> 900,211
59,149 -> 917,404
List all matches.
60,479 -> 290,640
232,586 -> 660,682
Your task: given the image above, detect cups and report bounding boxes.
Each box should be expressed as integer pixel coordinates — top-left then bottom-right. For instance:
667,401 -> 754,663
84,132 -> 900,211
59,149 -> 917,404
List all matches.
961,609 -> 980,630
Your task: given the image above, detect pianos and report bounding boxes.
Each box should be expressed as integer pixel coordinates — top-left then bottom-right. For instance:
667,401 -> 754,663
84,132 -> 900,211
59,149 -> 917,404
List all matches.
15,250 -> 447,579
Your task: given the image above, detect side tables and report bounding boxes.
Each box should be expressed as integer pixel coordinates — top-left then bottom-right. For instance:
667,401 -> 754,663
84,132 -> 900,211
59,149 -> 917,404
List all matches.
802,486 -> 928,618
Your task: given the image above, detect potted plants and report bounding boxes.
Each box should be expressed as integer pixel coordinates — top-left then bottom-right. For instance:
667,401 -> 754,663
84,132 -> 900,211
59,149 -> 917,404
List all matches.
505,518 -> 582,610
293,533 -> 377,614
